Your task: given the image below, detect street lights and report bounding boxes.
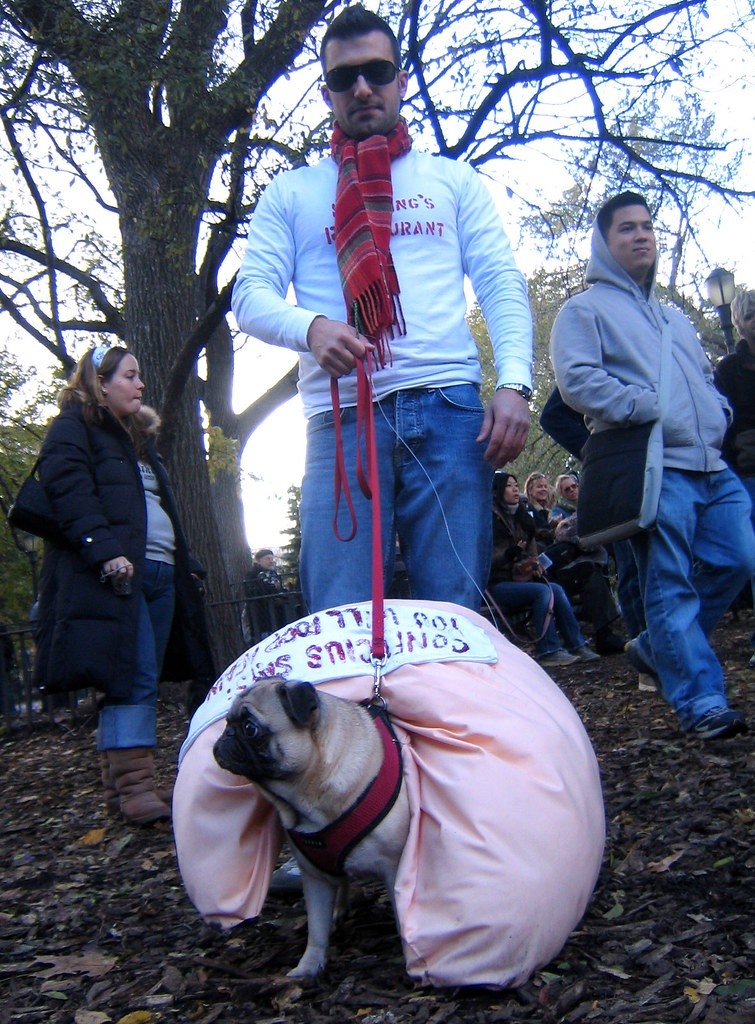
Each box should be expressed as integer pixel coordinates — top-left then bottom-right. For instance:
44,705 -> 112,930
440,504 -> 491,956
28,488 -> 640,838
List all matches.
704,267 -> 737,354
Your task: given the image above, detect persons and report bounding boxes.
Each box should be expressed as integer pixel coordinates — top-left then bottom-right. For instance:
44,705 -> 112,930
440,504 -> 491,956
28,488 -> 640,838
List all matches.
486,471 -> 621,665
0,622 -> 16,714
39,346 -> 207,826
243,550 -> 309,647
551,190 -> 755,745
714,290 -> 755,666
231,6 -> 534,895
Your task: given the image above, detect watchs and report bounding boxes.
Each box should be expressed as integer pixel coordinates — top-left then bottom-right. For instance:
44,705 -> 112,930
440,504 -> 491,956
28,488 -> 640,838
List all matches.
497,384 -> 532,401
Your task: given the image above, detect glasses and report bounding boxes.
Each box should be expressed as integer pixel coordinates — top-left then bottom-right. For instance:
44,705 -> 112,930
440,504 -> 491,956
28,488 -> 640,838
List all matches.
530,474 -> 546,484
322,58 -> 403,92
742,313 -> 755,321
563,484 -> 576,495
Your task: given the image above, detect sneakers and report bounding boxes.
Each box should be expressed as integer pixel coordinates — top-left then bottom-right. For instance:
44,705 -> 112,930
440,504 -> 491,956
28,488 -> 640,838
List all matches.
535,650 -> 579,667
685,704 -> 744,739
625,639 -> 659,683
572,646 -> 600,663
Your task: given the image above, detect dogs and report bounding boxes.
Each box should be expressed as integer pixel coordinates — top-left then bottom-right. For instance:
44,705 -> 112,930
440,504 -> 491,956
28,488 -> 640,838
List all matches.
213,677 -> 411,980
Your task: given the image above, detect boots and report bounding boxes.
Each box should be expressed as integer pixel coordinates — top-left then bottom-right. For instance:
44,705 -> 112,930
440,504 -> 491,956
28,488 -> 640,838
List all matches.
100,752 -> 172,813
108,746 -> 171,824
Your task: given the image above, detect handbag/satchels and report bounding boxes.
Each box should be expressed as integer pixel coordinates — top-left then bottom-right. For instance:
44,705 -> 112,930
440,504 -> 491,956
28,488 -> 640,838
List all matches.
6,418 -> 91,545
574,417 -> 664,548
554,514 -> 609,570
541,540 -> 592,579
512,556 -> 544,583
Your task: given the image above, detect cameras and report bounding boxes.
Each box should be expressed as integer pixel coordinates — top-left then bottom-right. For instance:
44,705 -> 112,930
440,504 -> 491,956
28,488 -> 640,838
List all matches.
112,573 -> 132,594
